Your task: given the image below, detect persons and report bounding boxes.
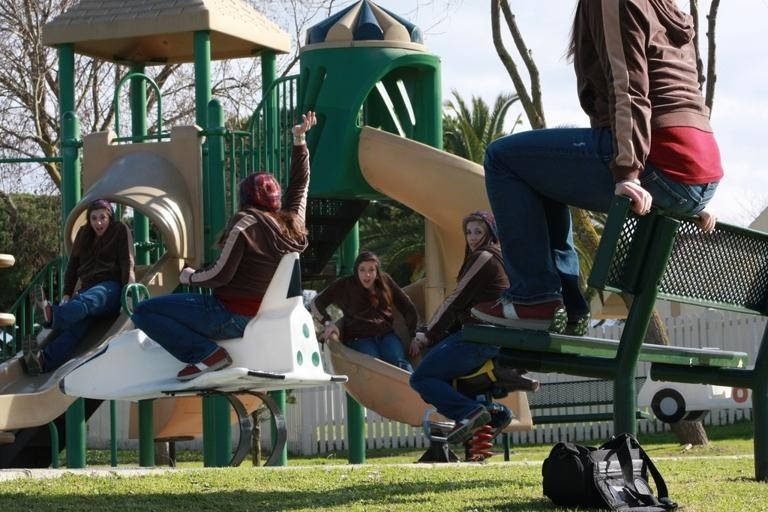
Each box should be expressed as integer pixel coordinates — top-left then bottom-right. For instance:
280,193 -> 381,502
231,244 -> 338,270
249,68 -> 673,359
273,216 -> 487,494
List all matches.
472,0 -> 724,330
22,200 -> 136,377
132,110 -> 318,381
310,252 -> 422,374
410,211 -> 511,444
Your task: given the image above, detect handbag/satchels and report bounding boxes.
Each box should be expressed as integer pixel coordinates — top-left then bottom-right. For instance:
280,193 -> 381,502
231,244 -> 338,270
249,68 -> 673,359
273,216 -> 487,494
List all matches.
543,431 -> 678,511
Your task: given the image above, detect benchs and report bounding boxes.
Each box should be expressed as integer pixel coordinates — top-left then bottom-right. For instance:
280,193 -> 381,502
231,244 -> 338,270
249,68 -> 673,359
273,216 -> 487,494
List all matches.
463,194 -> 766,484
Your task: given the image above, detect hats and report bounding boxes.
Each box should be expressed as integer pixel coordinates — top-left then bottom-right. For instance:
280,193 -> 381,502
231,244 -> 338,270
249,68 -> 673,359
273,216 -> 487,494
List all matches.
462,209 -> 499,244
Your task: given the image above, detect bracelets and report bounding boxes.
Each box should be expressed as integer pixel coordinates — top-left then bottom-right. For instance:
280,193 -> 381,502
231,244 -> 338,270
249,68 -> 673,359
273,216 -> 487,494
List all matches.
294,135 -> 306,141
59,299 -> 68,304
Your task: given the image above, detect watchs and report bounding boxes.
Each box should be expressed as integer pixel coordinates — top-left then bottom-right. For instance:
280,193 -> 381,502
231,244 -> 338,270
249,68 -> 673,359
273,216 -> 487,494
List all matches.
613,178 -> 641,186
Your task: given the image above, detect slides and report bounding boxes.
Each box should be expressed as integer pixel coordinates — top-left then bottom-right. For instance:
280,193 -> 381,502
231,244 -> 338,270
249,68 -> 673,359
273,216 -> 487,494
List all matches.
323,125 -> 534,429
1,126 -> 204,429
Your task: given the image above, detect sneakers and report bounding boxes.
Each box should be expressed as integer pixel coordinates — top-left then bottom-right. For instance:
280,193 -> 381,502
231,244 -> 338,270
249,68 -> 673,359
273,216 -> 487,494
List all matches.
487,401 -> 513,442
22,332 -> 43,375
177,346 -> 232,380
565,312 -> 590,334
470,297 -> 555,333
30,284 -> 52,326
447,403 -> 492,444
